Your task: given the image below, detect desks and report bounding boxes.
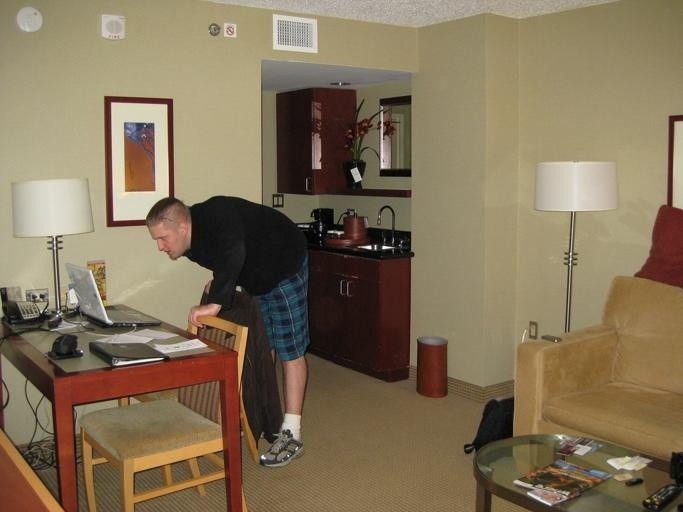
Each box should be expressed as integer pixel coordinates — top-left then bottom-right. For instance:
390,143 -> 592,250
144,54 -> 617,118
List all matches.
0,304 -> 243,511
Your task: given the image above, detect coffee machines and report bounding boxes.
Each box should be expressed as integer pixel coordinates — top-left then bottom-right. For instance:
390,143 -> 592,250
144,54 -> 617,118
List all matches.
308,207 -> 334,241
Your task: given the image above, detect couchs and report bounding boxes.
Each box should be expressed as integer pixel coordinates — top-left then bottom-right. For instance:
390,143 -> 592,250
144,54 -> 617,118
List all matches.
513,274 -> 682,465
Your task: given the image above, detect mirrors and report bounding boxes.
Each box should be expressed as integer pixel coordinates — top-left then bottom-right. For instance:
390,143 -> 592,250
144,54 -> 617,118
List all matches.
377,97 -> 413,177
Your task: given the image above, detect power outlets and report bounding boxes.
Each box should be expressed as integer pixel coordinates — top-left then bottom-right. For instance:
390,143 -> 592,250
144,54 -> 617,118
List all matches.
24,289 -> 49,303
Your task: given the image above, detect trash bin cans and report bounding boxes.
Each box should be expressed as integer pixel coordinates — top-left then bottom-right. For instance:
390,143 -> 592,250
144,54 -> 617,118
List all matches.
417,337 -> 448,398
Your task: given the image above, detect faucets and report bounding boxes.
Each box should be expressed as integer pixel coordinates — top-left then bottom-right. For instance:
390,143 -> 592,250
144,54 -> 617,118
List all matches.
377,204 -> 397,246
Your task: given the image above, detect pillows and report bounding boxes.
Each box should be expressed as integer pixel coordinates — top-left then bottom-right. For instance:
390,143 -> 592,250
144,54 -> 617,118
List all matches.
635,204 -> 682,288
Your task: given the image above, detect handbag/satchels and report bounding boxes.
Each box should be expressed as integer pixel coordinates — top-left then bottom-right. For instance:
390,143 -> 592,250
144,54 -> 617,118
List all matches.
473,397 -> 514,451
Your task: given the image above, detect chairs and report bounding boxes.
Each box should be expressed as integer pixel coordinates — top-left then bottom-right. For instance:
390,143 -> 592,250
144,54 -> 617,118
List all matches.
118,279 -> 264,495
78,313 -> 248,512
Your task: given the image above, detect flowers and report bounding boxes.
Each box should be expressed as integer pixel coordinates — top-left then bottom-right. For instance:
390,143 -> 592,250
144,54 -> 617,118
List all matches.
309,99 -> 398,164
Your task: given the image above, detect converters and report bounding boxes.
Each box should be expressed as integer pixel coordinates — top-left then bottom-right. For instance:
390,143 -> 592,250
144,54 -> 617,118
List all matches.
48,316 -> 62,328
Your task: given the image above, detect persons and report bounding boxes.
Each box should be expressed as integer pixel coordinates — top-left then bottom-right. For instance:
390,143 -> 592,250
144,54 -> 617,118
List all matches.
145,195 -> 312,469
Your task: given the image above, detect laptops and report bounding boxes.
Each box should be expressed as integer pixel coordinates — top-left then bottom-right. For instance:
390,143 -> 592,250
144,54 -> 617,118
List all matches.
65,264 -> 161,329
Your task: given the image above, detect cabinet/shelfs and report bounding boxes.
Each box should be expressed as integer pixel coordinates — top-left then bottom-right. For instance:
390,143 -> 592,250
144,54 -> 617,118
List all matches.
312,250 -> 408,380
274,88 -> 355,195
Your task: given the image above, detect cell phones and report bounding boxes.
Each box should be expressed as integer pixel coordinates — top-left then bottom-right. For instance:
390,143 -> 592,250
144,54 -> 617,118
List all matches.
541,335 -> 562,342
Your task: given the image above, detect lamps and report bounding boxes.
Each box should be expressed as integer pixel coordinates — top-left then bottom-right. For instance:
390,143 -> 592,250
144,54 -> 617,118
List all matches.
533,158 -> 619,334
10,177 -> 94,317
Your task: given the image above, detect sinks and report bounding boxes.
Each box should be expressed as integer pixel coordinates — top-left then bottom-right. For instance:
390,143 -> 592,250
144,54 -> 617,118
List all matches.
353,242 -> 398,254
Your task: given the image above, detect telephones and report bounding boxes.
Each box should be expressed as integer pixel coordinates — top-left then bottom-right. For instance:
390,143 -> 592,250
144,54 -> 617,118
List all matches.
2,300 -> 41,323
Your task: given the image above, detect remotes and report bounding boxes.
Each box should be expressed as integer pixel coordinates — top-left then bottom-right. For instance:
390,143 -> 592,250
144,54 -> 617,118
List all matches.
643,484 -> 681,510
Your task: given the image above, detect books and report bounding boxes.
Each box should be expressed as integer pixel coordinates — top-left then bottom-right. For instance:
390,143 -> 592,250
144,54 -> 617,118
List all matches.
514,459 -> 613,499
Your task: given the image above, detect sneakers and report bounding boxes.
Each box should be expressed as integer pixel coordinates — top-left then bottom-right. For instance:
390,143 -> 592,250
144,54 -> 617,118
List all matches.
261,429 -> 304,467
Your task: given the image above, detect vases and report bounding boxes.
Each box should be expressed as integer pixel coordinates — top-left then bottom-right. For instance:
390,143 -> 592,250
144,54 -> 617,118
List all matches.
341,159 -> 364,187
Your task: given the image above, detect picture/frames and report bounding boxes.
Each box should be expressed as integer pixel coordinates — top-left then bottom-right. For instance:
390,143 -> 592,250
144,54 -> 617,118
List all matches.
666,115 -> 683,210
103,94 -> 174,227
